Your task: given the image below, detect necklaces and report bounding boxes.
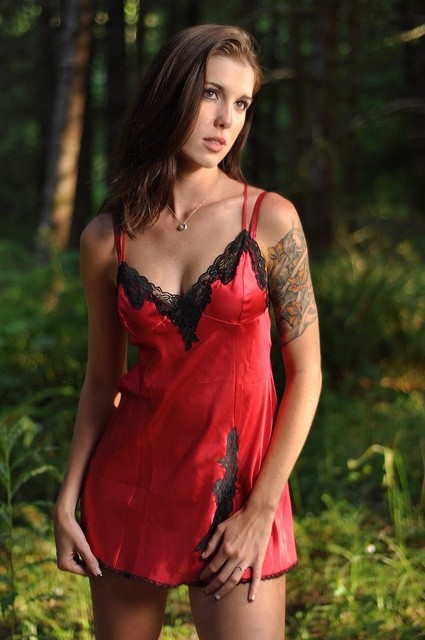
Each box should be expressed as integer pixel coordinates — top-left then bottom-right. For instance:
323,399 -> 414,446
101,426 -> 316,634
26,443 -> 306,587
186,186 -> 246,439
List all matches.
167,186 -> 216,231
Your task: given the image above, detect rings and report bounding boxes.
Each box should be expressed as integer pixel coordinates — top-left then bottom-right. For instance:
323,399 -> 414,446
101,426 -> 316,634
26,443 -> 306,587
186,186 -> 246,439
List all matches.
236,567 -> 247,572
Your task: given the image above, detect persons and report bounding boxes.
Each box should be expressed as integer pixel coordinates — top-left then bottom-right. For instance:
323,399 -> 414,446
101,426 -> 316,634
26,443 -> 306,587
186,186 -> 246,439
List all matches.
55,24 -> 322,640
